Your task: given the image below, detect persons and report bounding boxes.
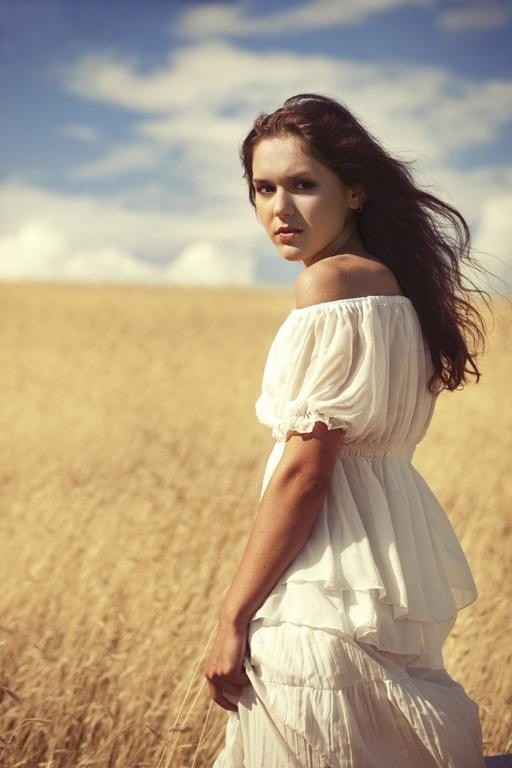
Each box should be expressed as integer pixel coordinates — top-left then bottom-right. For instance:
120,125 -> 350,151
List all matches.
200,93 -> 511,768
